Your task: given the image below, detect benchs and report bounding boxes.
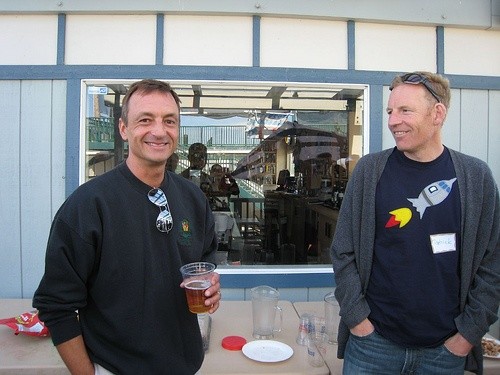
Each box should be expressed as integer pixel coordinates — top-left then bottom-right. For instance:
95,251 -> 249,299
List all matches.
230,197 -> 266,244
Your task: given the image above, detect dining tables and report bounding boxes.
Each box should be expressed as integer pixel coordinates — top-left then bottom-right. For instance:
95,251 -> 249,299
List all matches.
0,298 -> 500,375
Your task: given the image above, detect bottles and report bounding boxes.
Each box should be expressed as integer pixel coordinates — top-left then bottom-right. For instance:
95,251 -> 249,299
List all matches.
332,185 -> 338,202
338,187 -> 344,206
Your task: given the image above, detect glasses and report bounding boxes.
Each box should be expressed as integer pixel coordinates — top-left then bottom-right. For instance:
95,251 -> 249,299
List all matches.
389,73 -> 440,103
147,185 -> 174,233
190,153 -> 207,160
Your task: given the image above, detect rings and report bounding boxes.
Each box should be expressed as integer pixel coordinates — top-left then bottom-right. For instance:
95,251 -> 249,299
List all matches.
218,291 -> 221,299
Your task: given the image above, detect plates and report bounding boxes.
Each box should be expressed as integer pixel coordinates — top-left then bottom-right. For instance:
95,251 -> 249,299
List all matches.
242,340 -> 293,363
481,336 -> 500,358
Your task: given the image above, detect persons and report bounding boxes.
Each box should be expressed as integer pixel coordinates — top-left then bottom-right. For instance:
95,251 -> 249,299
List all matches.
331,72 -> 500,375
179,143 -> 240,250
32,79 -> 221,375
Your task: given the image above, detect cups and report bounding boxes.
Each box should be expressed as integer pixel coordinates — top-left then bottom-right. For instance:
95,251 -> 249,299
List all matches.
251,286 -> 282,340
180,262 -> 215,315
306,332 -> 329,367
295,314 -> 316,346
324,290 -> 341,345
197,315 -> 211,351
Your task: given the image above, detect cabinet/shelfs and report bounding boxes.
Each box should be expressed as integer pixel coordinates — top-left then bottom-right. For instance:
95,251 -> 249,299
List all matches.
264,190 -> 350,264
